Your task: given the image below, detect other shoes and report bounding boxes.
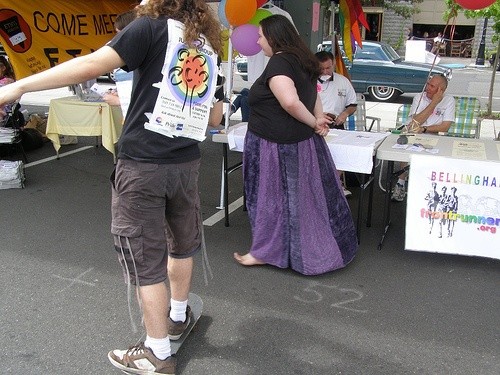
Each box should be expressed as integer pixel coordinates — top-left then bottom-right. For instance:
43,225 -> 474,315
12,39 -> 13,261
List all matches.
221,116 -> 230,127
382,185 -> 404,201
342,186 -> 352,198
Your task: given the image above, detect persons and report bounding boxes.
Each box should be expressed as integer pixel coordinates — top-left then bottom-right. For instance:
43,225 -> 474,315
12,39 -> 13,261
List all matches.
313,51 -> 358,197
234,14 -> 358,276
0,0 -> 230,375
392,72 -> 456,204
433,32 -> 446,57
0,55 -> 16,125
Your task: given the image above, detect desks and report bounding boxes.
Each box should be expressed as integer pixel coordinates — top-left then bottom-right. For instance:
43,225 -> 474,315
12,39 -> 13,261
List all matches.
211,122 -> 392,245
376,132 -> 500,251
45,94 -> 123,165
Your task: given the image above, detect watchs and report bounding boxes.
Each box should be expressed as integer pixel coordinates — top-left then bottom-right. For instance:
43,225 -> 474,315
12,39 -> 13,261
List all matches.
423,126 -> 427,133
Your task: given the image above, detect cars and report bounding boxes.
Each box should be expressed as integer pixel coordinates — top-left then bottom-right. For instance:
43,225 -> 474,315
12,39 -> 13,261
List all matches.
234,40 -> 452,103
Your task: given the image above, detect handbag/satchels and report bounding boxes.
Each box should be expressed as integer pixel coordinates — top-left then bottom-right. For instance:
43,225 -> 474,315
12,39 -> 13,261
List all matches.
0,111 -> 78,157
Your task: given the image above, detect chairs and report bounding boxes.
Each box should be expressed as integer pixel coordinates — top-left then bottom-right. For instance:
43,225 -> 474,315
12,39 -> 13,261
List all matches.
340,92 -> 387,192
436,40 -> 447,56
0,94 -> 29,164
387,96 -> 482,180
450,41 -> 462,57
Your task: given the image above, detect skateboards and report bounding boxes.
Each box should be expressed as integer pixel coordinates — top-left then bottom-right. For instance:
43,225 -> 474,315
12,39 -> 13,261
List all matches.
120,292 -> 202,375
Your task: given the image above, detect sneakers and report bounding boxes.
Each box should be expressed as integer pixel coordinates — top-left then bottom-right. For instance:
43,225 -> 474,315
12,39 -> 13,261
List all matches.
107,343 -> 178,375
168,305 -> 191,340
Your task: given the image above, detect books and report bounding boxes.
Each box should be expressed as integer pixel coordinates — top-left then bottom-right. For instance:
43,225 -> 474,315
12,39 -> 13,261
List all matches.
0,128 -> 21,144
0,160 -> 26,190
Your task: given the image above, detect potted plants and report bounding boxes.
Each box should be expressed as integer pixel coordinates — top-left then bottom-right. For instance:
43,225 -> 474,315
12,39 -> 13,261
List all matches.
485,47 -> 498,66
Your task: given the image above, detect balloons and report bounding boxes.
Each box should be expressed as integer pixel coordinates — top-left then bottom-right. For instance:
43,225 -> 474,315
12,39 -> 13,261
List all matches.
454,0 -> 497,10
216,0 -> 274,56
